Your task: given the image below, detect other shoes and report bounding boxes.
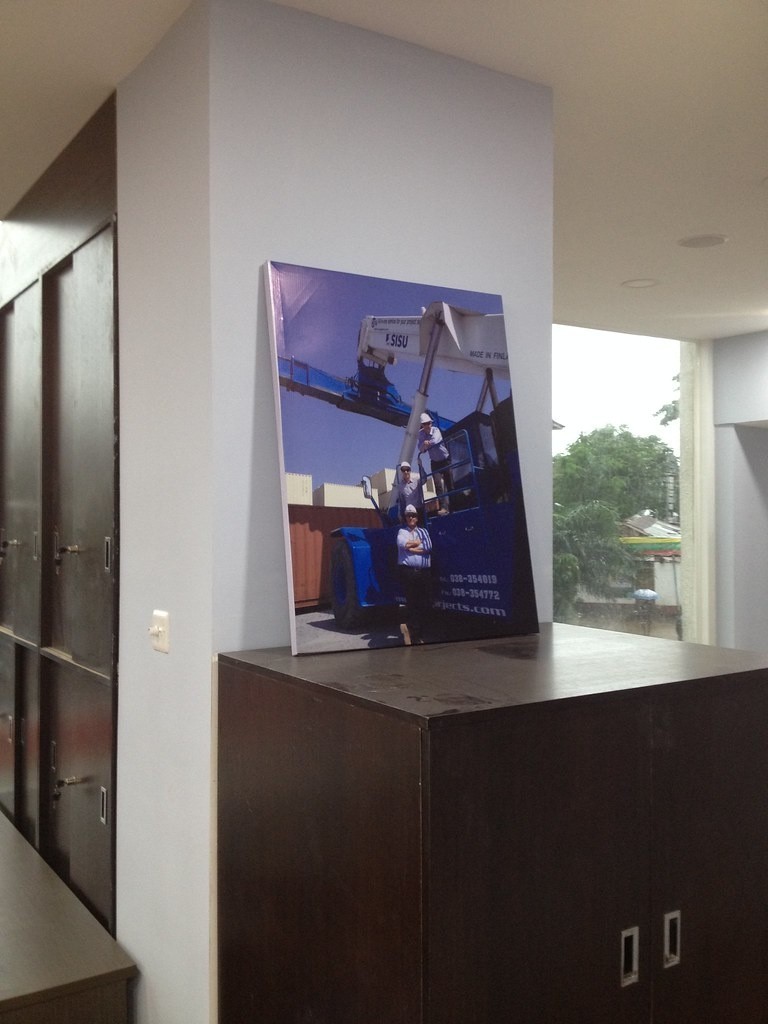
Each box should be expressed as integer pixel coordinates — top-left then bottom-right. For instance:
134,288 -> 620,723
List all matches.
400,624 -> 411,644
438,509 -> 448,515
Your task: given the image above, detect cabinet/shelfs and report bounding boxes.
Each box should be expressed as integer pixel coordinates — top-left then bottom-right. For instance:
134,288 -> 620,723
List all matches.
217,622 -> 768,1024
0,637 -> 117,937
0,211 -> 116,682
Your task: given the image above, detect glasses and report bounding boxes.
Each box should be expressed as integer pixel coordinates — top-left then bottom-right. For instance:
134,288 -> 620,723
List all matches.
424,422 -> 430,425
406,513 -> 417,518
402,468 -> 410,472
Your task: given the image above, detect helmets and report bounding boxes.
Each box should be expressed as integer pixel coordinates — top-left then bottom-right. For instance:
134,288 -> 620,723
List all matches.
404,504 -> 418,513
420,413 -> 434,424
400,462 -> 411,469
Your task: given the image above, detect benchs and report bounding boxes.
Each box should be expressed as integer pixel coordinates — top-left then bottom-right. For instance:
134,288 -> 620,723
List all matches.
0,810 -> 138,1024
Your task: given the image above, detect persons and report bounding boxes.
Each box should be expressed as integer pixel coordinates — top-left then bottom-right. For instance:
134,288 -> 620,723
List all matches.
418,411 -> 459,515
639,600 -> 652,636
397,458 -> 432,645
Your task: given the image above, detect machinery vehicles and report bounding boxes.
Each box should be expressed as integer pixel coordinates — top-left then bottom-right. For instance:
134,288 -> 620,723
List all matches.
276,299 -> 539,640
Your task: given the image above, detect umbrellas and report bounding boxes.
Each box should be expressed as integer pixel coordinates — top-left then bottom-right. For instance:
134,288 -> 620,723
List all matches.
632,589 -> 658,600
